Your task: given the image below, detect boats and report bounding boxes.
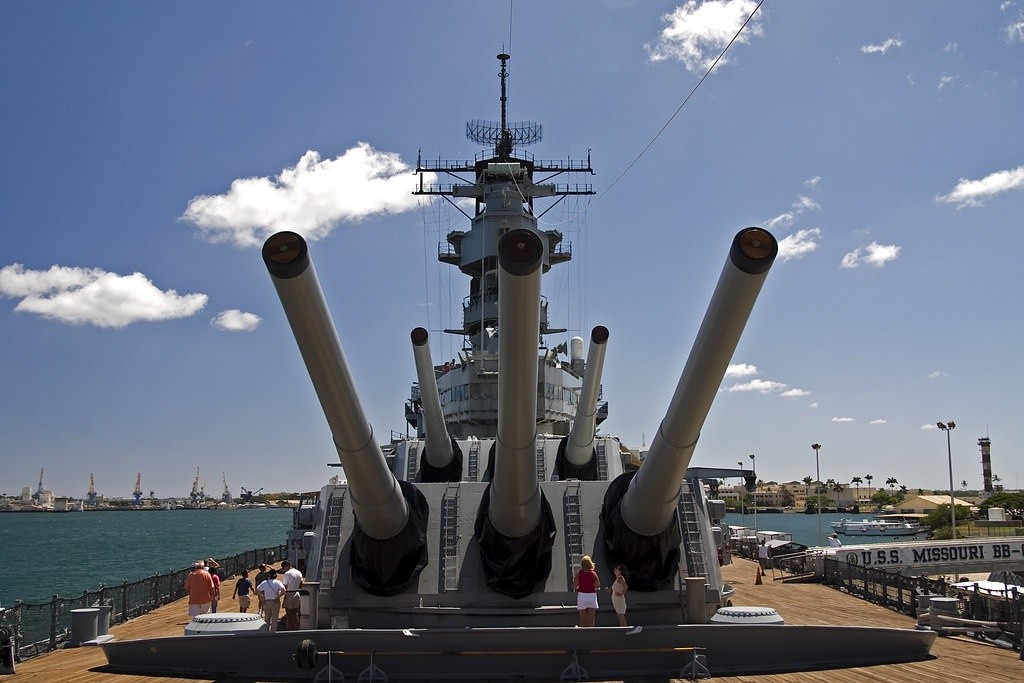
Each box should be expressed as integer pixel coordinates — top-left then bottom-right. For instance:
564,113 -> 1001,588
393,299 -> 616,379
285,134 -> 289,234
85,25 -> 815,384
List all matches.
829,516 -> 920,537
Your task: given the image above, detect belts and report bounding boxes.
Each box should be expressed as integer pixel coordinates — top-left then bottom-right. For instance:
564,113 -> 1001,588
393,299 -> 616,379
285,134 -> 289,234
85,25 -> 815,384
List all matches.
285,590 -> 293,592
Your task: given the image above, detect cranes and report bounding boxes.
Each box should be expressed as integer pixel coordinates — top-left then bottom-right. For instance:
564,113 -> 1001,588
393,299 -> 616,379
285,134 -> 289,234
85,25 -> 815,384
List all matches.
189,466 -> 207,507
33,466 -> 47,505
130,473 -> 144,508
85,472 -> 98,509
220,471 -> 233,504
238,486 -> 264,504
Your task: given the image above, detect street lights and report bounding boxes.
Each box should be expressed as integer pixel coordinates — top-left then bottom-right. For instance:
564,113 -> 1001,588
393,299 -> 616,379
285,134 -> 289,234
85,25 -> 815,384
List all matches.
810,444 -> 824,548
749,455 -> 758,536
737,460 -> 745,527
936,420 -> 959,583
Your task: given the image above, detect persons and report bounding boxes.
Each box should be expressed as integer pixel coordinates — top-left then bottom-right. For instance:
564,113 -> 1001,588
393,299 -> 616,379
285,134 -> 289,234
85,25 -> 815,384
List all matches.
281,560 -> 303,631
573,555 -> 600,627
257,569 -> 286,631
255,563 -> 268,618
441,359 -> 455,372
184,557 -> 220,622
758,540 -> 768,576
232,570 -> 255,612
826,532 -> 841,547
611,566 -> 628,625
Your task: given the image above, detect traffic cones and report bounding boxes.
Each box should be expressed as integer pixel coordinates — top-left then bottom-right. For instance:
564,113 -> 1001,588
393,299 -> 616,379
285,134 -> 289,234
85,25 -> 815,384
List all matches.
754,567 -> 763,586
